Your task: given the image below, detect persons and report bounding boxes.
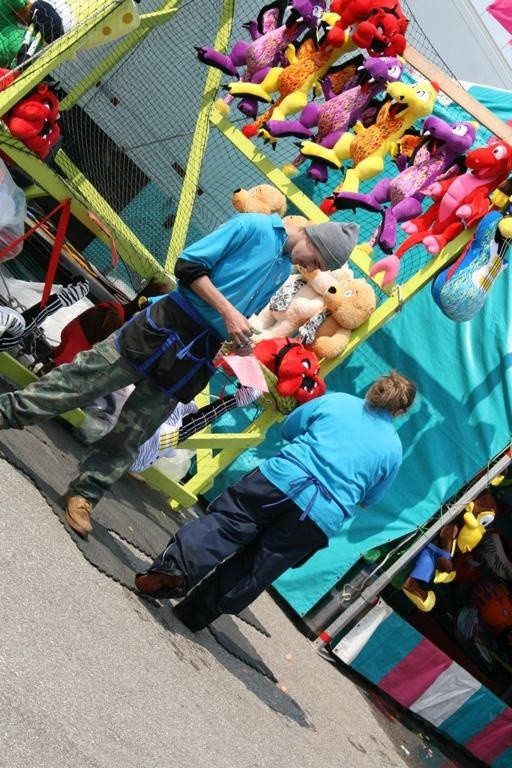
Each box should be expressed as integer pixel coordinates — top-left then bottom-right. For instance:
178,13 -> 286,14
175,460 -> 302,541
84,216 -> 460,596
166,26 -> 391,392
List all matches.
129,369 -> 422,633
1,211 -> 361,537
184,1 -> 512,283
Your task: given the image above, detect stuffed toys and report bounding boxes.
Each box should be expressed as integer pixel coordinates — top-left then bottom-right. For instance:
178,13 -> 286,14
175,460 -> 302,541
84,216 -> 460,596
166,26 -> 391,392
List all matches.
183,1 -> 512,283
193,175 -> 378,417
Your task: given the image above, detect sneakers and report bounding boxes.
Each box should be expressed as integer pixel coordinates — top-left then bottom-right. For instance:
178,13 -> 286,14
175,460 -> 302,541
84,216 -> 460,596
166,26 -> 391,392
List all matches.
134,572 -> 186,599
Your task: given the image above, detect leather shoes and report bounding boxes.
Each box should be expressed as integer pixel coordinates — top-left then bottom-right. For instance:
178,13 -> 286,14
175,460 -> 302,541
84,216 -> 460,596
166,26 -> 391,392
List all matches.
59,492 -> 93,538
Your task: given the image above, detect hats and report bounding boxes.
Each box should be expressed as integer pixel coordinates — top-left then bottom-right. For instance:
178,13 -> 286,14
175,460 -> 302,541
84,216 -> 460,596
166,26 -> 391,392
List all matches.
305,221 -> 361,272
